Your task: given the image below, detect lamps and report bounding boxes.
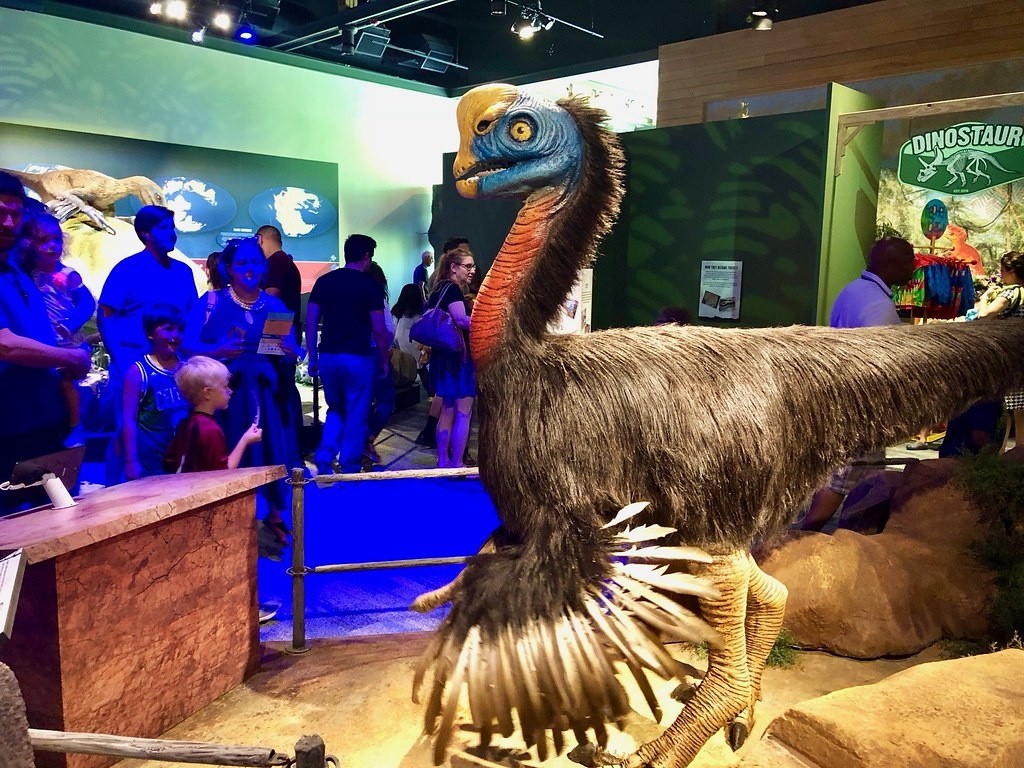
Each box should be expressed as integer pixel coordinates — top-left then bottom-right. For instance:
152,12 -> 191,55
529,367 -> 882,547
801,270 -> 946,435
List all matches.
490,0 -> 553,40
751,0 -> 773,31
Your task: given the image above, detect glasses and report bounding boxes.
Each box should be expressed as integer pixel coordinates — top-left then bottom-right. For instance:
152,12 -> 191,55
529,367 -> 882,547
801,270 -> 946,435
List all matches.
456,263 -> 477,271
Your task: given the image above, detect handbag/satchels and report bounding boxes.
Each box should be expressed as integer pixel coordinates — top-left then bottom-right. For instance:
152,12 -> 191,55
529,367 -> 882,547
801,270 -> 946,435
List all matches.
409,282 -> 466,354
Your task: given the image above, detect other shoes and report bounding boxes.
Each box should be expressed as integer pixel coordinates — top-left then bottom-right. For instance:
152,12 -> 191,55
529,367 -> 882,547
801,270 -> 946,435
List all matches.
363,439 -> 380,463
415,432 -> 437,449
462,450 -> 477,469
427,394 -> 437,403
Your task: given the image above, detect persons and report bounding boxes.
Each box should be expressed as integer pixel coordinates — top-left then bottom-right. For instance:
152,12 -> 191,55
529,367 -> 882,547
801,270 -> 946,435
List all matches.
161,355 -> 276,622
977,251 -> 1024,455
0,172 -> 478,534
792,237 -> 916,533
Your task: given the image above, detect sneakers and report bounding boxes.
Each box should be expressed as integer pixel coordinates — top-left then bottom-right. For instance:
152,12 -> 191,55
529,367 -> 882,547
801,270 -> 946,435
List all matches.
906,441 -> 928,450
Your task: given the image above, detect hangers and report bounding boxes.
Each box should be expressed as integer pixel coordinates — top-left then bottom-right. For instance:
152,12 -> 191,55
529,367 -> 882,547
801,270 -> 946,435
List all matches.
912,247 -> 969,270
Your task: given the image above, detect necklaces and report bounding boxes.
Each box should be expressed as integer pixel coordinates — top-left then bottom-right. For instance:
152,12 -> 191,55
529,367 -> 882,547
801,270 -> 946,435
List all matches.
229,285 -> 264,310
